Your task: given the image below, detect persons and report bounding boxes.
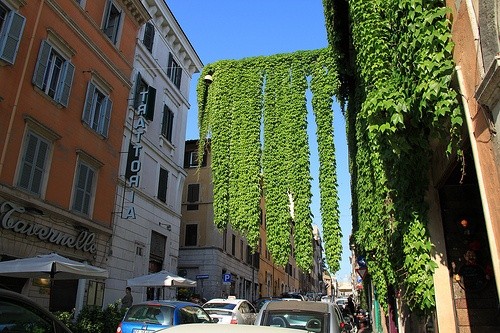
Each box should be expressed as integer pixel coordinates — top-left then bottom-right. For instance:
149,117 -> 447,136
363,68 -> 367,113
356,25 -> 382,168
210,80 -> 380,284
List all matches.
121,287 -> 133,307
345,297 -> 354,322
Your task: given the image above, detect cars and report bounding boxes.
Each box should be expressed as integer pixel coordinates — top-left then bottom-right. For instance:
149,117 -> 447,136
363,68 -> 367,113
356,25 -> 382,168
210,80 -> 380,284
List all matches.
153,320 -> 317,333
0,290 -> 71,333
115,296 -> 259,333
250,292 -> 352,333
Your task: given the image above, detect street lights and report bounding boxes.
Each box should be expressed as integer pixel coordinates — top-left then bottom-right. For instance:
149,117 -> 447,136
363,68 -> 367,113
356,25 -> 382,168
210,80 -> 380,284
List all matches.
252,242 -> 258,304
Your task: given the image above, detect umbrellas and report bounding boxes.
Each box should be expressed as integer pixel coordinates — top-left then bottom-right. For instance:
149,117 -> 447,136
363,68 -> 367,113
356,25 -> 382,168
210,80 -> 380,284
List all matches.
0,254 -> 108,312
128,269 -> 197,300
